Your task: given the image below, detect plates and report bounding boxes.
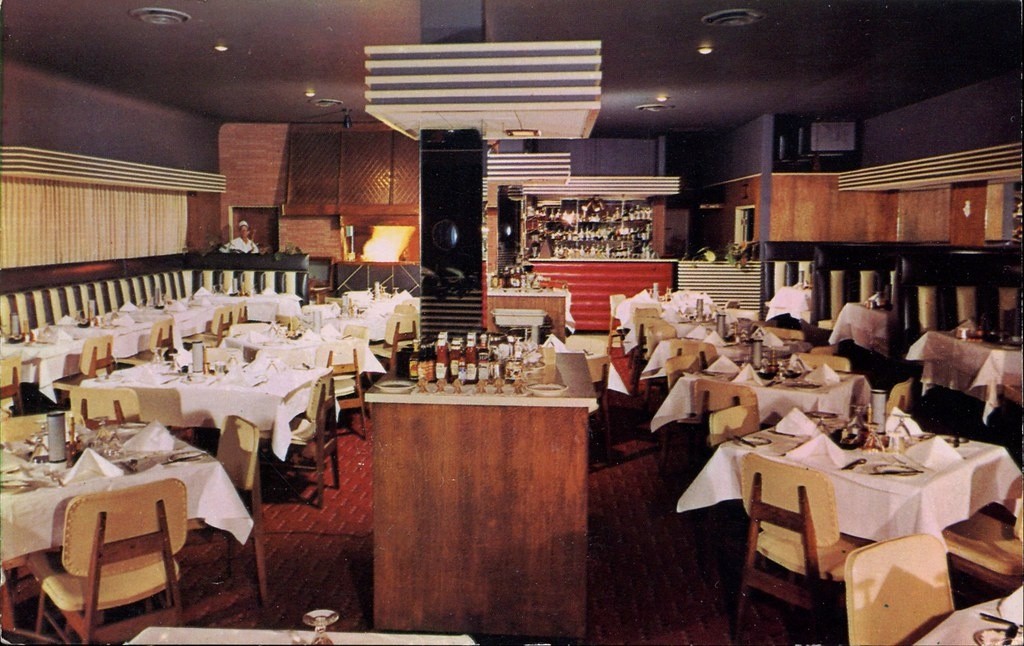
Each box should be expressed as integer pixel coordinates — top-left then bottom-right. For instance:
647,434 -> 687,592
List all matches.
735,436 -> 773,448
918,434 -> 970,447
168,450 -> 208,465
783,380 -> 822,391
373,380 -> 416,393
972,628 -> 1024,646
525,383 -> 568,396
873,464 -> 920,479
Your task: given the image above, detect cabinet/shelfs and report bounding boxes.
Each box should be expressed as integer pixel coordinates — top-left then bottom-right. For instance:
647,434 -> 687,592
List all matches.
537,219 -> 653,243
364,353 -> 600,646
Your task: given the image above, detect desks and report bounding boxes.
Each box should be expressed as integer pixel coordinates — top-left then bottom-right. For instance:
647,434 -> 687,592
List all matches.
79,350 -> 341,461
125,625 -> 475,646
905,331 -> 1022,425
912,598 -> 1024,646
0,410 -> 253,565
639,339 -> 812,382
487,287 -> 576,334
214,293 -> 303,322
615,290 -> 718,329
217,325 -> 388,380
337,261 -> 421,294
675,416 -> 1024,552
828,302 -> 897,358
765,286 -> 812,322
623,314 -> 739,355
309,316 -> 388,342
42,319 -> 184,358
137,305 -> 207,338
0,330 -> 87,404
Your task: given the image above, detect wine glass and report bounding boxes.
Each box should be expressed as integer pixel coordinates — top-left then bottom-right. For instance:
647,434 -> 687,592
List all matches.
264,355 -> 279,374
168,353 -> 182,370
29,432 -> 49,464
150,346 -> 161,364
92,416 -> 109,445
302,609 -> 340,646
224,349 -> 240,370
103,425 -> 126,459
32,419 -> 48,437
891,413 -> 912,444
861,421 -> 885,455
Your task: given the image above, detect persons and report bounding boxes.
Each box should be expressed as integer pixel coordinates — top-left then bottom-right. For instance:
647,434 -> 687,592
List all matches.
219,220 -> 259,253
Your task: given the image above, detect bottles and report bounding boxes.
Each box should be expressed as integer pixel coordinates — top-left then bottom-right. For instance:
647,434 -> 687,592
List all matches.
499,266 -> 521,288
550,205 -> 655,259
408,331 -> 524,384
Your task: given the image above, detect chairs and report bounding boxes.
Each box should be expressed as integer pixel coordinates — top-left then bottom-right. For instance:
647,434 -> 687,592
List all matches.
69,386 -> 141,425
789,351 -> 852,443
51,333 -> 115,391
843,533 -> 954,646
941,474 -> 1024,592
693,378 -> 759,464
606,294 -> 627,357
999,383 -> 1024,443
117,300 -> 421,510
187,414 -> 269,602
668,338 -> 718,369
658,355 -> 706,483
632,317 -> 669,394
644,325 -> 678,410
734,455 -> 856,646
885,377 -> 913,414
35,478 -> 188,646
708,402 -> 760,453
626,307 -> 658,369
764,326 -> 805,340
0,350 -> 24,417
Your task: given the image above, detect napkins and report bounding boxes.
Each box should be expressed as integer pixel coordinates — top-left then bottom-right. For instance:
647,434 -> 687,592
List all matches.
394,290 -> 412,303
725,311 -> 739,323
885,407 -> 923,438
905,435 -> 962,472
244,350 -> 291,373
123,365 -> 162,387
704,334 -> 841,387
177,346 -> 194,365
634,289 -> 651,300
298,329 -> 320,341
783,432 -> 848,472
703,330 -> 727,347
120,420 -> 175,451
776,407 -> 817,436
245,329 -> 270,343
319,322 -> 341,338
950,318 -> 977,335
661,307 -> 683,322
60,447 -> 124,484
686,325 -> 706,341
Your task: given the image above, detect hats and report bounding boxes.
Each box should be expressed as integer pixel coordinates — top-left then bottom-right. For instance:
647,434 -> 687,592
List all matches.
239,220 -> 248,228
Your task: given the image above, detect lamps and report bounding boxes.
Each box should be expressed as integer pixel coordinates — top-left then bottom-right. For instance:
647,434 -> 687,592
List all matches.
342,115 -> 352,129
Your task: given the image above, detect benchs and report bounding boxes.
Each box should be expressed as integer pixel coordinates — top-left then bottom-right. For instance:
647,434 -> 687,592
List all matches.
0,269 -> 309,335
